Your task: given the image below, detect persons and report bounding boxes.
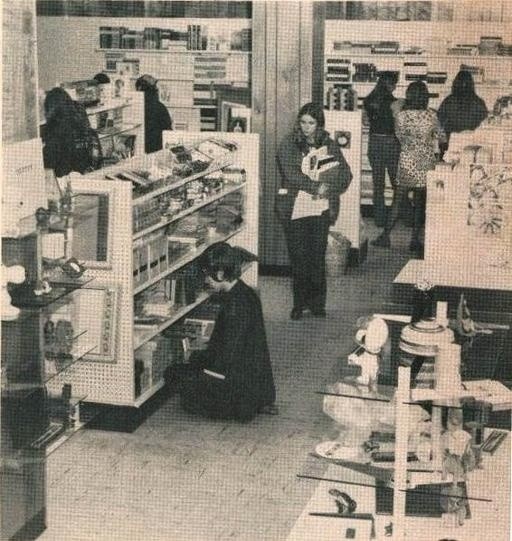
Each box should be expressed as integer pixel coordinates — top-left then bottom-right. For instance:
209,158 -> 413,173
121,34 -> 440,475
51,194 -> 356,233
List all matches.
274,103 -> 354,320
370,81 -> 449,253
94,73 -> 111,83
162,241 -> 280,421
438,70 -> 489,151
135,73 -> 174,154
364,70 -> 416,227
42,86 -> 94,177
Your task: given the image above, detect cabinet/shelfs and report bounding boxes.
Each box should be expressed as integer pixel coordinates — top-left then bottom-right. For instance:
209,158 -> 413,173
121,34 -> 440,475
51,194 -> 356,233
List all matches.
321,50 -> 512,206
39,48 -> 253,152
2,207 -> 98,478
46,130 -> 261,435
295,309 -> 507,537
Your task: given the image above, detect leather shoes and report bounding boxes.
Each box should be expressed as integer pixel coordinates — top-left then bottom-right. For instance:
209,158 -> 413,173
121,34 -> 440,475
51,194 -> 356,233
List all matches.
290,307 -> 326,318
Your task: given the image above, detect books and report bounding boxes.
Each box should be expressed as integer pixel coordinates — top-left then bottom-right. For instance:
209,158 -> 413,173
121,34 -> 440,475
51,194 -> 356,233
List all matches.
312,154 -> 340,196
99,24 -> 204,50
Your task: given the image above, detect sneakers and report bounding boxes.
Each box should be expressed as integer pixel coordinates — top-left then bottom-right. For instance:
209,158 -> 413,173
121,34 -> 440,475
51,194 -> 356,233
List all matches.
410,240 -> 422,250
371,235 -> 391,247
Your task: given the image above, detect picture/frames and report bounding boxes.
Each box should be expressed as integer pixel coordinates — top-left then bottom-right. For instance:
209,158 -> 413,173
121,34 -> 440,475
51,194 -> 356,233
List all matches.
43,279 -> 119,364
64,188 -> 114,267
